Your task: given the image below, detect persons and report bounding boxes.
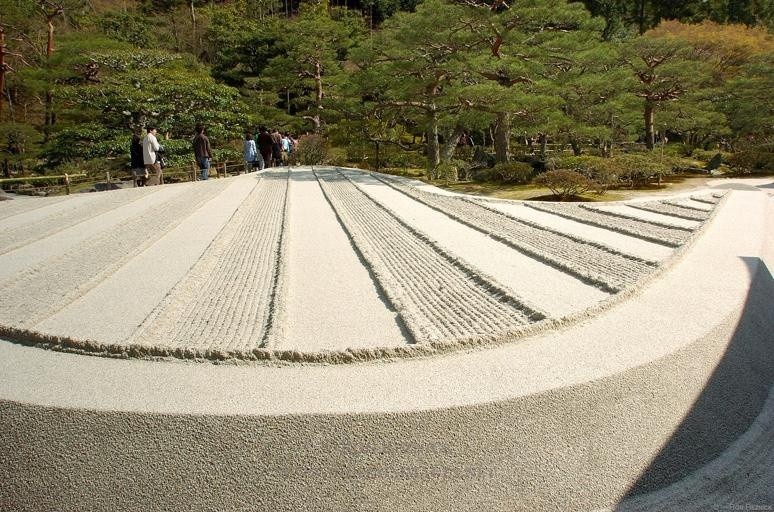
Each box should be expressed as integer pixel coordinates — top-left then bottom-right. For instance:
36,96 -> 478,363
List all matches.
193,125 -> 212,180
244,127 -> 297,171
130,127 -> 164,187
